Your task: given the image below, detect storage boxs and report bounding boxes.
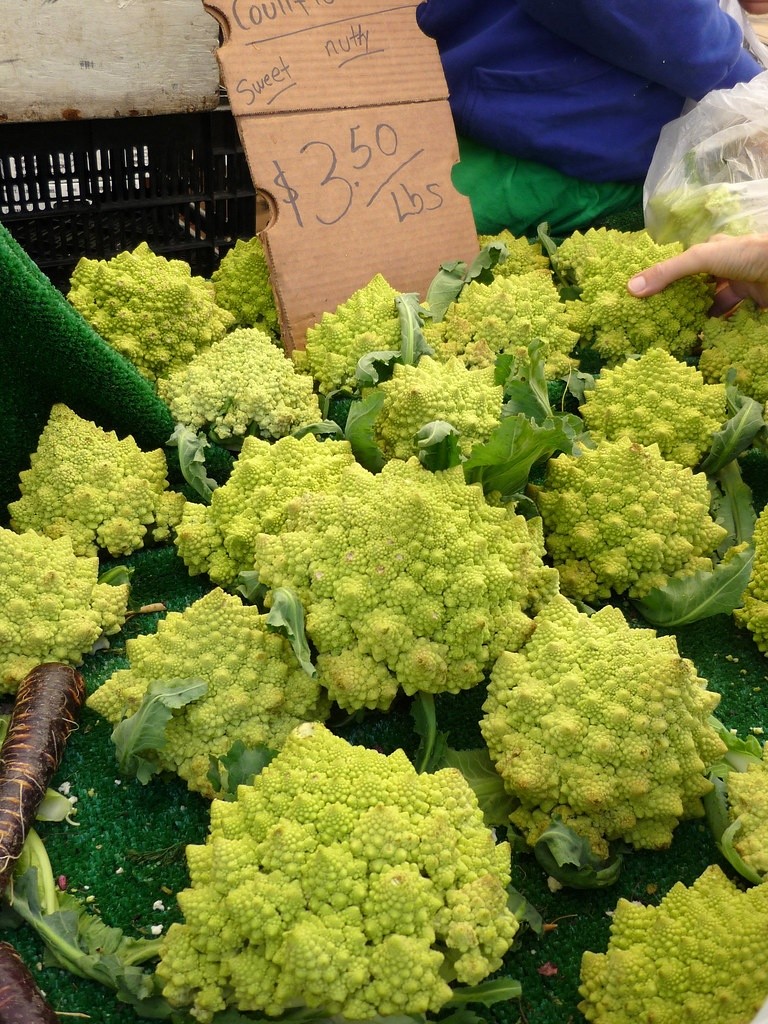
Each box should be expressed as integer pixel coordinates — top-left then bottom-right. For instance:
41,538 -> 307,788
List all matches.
0,101 -> 258,296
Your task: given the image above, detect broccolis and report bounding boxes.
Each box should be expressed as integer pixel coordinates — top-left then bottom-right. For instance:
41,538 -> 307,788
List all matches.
0,183 -> 768,1023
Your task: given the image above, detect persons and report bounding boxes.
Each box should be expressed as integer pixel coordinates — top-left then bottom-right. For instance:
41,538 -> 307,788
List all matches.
414,0 -> 768,235
627,233 -> 768,318
